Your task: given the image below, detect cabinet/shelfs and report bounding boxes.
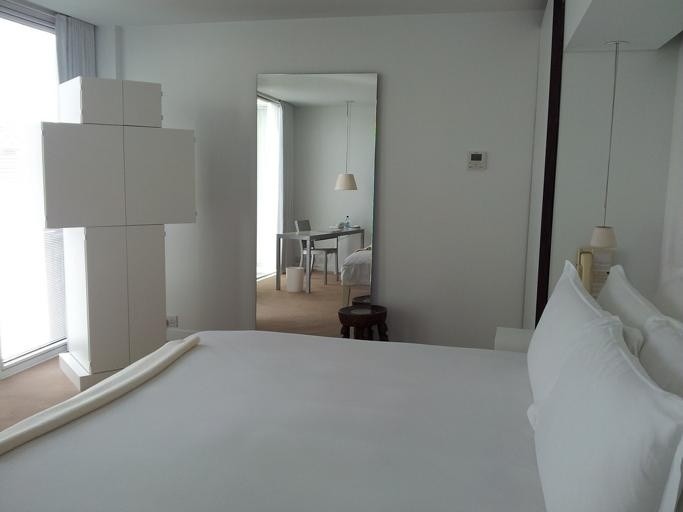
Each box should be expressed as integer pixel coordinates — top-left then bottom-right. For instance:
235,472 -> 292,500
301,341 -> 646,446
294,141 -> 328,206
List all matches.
30,75 -> 197,393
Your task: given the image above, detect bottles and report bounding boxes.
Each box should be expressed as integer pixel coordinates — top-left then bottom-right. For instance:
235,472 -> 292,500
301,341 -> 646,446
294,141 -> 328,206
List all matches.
345,215 -> 351,227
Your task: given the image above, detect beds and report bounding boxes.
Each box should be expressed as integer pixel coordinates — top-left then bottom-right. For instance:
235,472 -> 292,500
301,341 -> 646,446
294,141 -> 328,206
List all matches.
1,328 -> 683,510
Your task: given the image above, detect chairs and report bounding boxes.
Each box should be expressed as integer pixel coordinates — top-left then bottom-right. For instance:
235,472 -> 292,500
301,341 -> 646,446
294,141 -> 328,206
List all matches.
294,220 -> 339,285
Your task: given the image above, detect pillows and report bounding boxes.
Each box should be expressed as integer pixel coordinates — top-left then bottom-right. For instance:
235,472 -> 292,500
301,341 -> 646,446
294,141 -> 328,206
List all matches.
526,260 -> 681,511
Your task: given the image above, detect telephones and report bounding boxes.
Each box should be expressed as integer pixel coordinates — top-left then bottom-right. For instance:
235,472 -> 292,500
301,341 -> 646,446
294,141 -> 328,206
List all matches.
336,221 -> 344,229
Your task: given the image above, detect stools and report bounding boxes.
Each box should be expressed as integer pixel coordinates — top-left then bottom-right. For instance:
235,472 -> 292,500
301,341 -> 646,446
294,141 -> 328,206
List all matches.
338,304 -> 389,341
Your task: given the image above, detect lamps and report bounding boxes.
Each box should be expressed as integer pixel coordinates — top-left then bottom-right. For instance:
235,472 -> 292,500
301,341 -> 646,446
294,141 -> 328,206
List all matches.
591,226 -> 617,263
334,102 -> 358,192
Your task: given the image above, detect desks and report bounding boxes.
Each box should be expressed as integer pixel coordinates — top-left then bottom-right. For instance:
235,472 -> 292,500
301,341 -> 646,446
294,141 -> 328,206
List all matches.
275,227 -> 364,294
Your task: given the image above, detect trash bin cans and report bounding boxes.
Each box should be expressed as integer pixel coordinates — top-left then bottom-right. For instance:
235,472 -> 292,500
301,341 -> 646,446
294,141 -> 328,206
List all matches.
286,267 -> 304,292
